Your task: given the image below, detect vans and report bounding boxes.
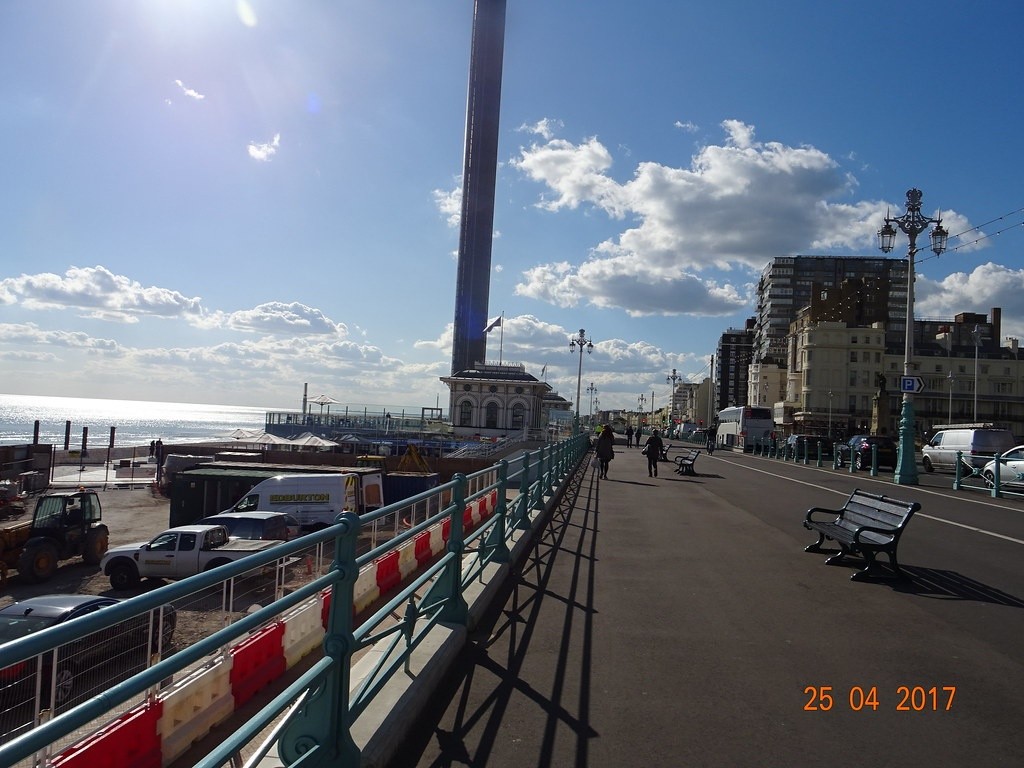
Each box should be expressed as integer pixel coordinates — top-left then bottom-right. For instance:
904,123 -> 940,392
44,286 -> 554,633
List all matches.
219,473 -> 386,544
921,428 -> 1016,478
192,512 -> 288,540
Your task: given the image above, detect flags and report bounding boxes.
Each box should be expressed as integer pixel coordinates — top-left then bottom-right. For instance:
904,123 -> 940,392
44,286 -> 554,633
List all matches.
541,364 -> 546,376
483,316 -> 501,333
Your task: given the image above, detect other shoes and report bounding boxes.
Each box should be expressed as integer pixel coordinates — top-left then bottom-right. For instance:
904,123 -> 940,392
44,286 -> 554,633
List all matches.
599,472 -> 603,479
604,477 -> 608,481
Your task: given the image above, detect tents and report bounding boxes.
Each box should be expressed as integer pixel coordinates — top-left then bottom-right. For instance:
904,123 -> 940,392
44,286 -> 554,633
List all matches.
229,429 -> 339,453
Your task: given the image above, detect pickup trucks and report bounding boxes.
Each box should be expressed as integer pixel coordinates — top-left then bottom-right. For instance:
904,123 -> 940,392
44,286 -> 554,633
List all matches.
99,524 -> 287,595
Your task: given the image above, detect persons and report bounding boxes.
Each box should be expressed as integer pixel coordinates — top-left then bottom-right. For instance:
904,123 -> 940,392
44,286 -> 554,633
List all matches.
386,412 -> 391,425
643,429 -> 663,477
635,429 -> 641,448
688,428 -> 692,432
149,438 -> 163,458
594,424 -> 615,480
704,426 -> 715,452
579,423 -> 585,432
626,426 -> 634,448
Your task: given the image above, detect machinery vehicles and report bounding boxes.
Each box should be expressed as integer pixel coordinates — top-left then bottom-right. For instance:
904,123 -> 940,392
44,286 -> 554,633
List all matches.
0,486 -> 110,591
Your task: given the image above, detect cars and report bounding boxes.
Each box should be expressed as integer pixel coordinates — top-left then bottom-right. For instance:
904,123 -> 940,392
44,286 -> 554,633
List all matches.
982,445 -> 1024,489
0,595 -> 176,720
781,435 -> 899,469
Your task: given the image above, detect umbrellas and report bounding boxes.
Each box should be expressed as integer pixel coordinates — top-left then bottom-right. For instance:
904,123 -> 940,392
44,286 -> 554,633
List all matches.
298,394 -> 343,414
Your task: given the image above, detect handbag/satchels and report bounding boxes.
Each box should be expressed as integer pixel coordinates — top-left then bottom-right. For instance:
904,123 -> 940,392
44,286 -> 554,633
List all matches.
642,444 -> 651,455
591,456 -> 601,468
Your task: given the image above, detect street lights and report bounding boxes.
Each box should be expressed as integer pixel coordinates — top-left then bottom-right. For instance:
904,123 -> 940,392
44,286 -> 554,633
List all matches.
877,187 -> 949,486
828,389 -> 834,437
587,383 -> 600,432
972,323 -> 982,422
666,369 -> 682,440
569,329 -> 593,436
637,394 -> 647,428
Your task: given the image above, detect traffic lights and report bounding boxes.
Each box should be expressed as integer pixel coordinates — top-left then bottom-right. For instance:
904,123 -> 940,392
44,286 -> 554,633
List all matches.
643,418 -> 645,423
663,416 -> 665,423
669,415 -> 672,423
700,420 -> 703,425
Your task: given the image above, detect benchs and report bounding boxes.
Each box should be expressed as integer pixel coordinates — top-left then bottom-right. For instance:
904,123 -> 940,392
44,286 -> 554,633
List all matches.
658,443 -> 672,462
802,488 -> 921,582
674,449 -> 701,476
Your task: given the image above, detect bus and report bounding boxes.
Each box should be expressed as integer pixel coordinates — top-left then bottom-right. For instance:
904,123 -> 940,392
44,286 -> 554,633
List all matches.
714,406 -> 774,450
